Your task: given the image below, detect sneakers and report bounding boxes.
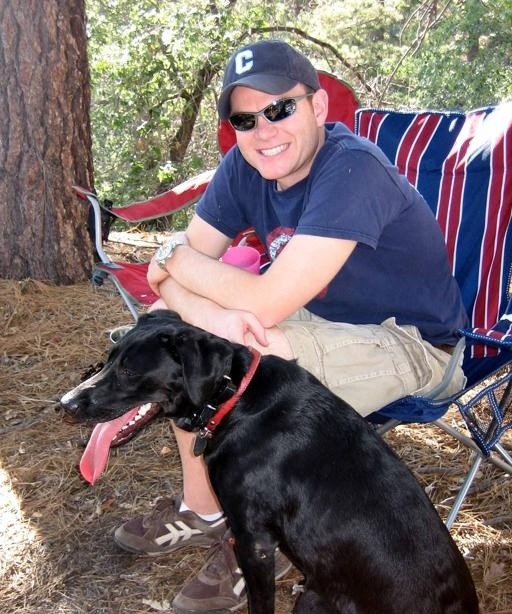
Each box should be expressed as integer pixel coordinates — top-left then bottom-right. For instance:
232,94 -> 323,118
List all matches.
113,493 -> 231,554
172,525 -> 293,614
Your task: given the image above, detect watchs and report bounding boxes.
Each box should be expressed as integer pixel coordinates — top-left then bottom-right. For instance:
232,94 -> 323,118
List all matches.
154,240 -> 188,269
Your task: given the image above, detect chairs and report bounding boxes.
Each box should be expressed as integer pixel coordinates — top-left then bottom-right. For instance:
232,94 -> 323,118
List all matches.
70,70 -> 364,327
260,103 -> 511,531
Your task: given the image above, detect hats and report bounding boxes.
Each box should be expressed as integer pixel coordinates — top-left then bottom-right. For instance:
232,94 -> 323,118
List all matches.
217,39 -> 320,117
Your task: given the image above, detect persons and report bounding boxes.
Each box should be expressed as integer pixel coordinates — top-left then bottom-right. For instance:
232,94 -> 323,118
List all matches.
114,39 -> 472,613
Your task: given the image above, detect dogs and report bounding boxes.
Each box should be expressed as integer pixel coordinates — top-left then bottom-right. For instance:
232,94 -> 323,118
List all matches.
59,308 -> 480,614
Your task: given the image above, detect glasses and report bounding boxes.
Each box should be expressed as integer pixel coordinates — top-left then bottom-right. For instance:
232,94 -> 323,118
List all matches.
228,93 -> 316,131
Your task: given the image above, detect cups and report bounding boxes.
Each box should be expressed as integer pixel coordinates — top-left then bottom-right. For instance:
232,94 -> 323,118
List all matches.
222,246 -> 261,274
